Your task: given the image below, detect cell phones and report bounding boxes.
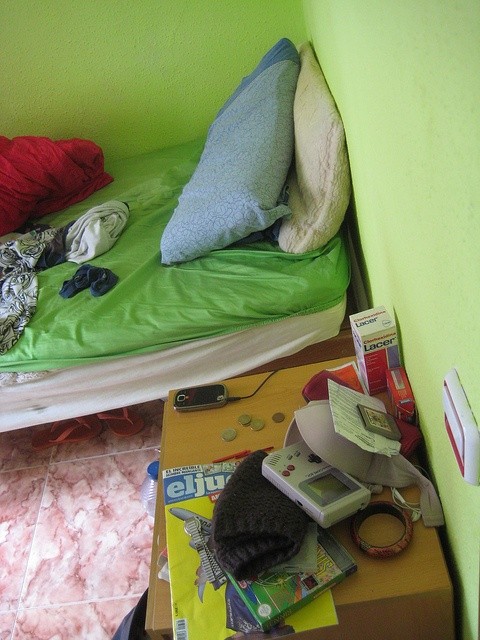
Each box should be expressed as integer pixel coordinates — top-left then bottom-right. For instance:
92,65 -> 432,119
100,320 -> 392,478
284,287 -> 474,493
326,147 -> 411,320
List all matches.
173,383 -> 228,413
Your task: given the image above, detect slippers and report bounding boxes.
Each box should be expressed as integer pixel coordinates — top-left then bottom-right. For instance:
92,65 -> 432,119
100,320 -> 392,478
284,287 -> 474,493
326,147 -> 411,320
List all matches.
31,416 -> 102,450
96,407 -> 145,437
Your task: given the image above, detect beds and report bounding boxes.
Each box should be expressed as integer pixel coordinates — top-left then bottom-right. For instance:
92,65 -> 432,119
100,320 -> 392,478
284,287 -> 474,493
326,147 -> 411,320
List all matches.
0,135 -> 379,432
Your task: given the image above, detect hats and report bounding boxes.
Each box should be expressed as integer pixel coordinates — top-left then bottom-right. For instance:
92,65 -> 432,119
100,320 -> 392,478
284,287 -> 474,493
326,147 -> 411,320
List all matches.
212,450 -> 309,581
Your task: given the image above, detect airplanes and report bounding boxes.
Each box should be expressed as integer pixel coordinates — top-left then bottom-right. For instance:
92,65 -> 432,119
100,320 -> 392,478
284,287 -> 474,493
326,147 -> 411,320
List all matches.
168,507 -> 216,604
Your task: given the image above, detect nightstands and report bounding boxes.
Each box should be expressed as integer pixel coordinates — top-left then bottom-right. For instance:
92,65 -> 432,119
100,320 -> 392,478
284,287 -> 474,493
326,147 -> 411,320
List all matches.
142,354 -> 458,639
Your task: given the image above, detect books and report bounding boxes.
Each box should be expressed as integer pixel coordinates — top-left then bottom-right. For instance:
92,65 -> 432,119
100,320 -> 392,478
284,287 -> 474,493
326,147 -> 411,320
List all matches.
161,461 -> 341,639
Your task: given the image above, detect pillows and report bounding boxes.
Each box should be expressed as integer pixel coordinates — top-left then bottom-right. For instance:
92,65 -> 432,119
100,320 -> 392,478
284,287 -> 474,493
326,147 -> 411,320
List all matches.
278,42 -> 354,256
159,35 -> 300,267
